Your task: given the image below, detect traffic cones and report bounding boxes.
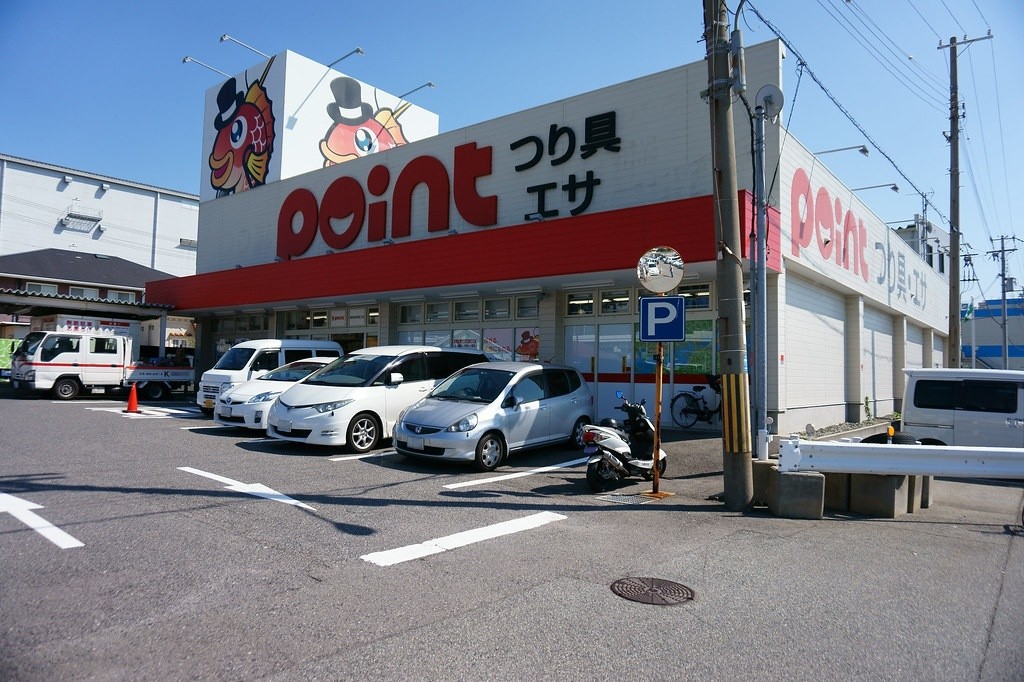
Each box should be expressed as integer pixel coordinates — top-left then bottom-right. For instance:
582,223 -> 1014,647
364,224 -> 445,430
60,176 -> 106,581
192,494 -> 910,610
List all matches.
122,383 -> 142,413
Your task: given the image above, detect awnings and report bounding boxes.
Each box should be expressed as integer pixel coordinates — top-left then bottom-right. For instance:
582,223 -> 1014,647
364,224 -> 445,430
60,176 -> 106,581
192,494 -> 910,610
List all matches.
0,289 -> 175,358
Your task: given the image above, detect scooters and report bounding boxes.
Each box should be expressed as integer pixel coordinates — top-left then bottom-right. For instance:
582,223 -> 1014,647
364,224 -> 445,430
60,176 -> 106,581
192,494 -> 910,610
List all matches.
580,389 -> 667,492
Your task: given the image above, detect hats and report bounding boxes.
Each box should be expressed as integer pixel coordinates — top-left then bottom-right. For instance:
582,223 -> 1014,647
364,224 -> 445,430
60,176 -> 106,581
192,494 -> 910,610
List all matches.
58,337 -> 70,341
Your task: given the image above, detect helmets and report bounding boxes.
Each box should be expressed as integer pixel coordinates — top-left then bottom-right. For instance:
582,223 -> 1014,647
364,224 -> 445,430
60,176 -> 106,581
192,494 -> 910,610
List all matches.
600,418 -> 617,427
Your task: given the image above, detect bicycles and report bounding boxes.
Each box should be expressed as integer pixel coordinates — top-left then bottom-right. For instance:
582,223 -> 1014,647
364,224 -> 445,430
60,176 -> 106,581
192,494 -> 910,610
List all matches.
669,381 -> 722,429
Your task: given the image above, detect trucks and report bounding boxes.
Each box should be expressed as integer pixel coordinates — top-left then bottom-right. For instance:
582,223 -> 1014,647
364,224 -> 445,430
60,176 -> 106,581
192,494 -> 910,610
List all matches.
10,330 -> 196,400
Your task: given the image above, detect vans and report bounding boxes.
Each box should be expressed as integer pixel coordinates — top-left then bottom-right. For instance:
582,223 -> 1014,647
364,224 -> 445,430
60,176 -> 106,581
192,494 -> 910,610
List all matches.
392,361 -> 595,472
266,346 -> 494,453
900,368 -> 1024,448
196,339 -> 345,416
213,357 -> 338,429
